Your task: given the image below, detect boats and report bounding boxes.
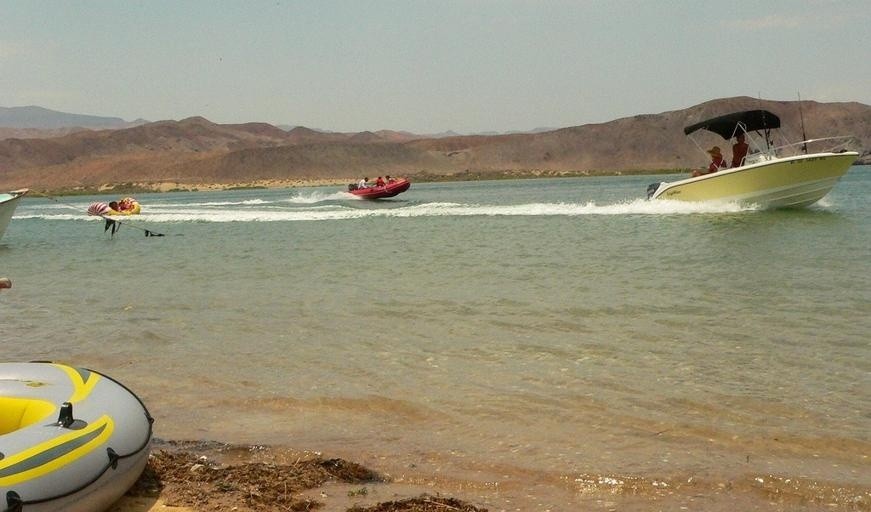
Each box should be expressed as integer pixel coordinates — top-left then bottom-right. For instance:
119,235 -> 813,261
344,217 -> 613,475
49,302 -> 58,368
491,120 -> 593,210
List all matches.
0,187 -> 26,245
87,197 -> 139,218
347,176 -> 410,198
634,90 -> 858,213
0,358 -> 155,512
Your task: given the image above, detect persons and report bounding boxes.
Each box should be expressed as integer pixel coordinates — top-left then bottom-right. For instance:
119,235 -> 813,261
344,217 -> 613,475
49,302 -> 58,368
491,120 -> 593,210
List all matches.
377,177 -> 385,186
0,277 -> 13,292
115,200 -> 126,233
693,146 -> 726,177
105,201 -> 118,239
384,175 -> 392,183
730,133 -> 759,168
358,176 -> 369,190
